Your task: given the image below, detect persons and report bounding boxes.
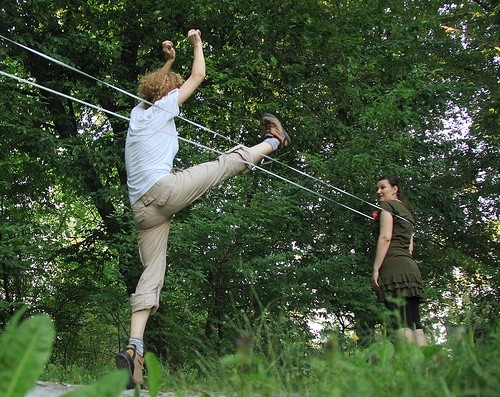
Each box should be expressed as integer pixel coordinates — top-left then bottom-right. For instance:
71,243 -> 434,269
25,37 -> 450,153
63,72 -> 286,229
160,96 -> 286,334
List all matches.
115,29 -> 293,390
371,174 -> 428,347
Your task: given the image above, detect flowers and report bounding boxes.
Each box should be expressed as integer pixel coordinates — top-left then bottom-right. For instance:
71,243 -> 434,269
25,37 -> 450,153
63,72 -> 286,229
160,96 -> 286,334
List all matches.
372,210 -> 379,221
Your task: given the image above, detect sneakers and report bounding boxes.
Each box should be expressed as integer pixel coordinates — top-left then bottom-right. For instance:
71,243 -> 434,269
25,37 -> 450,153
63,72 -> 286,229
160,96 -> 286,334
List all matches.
262,113 -> 292,150
116,344 -> 144,389
